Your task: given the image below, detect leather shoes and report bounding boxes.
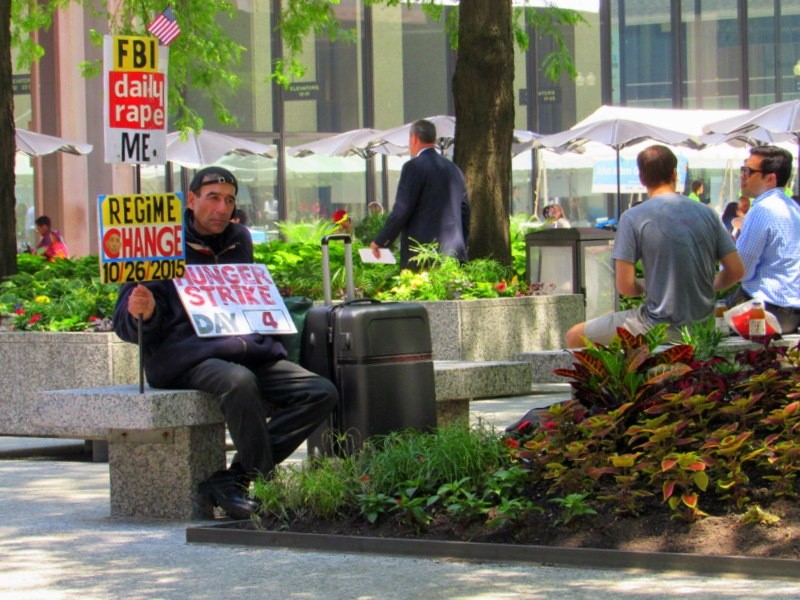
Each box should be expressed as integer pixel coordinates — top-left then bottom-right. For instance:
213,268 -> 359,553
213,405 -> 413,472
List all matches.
197,470 -> 264,520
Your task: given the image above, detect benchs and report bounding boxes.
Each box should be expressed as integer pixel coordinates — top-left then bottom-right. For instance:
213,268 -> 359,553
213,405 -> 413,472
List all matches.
506,330 -> 800,400
31,359 -> 532,520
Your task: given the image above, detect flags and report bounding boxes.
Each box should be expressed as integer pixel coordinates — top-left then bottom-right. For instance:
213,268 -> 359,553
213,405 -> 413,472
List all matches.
146,4 -> 181,46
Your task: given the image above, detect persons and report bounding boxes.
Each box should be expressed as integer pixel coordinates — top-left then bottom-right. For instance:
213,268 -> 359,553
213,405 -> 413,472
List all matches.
333,208 -> 351,234
26,215 -> 70,261
371,119 -> 471,275
564,145 -> 744,350
541,205 -> 570,229
230,209 -> 247,224
112,166 -> 340,517
687,146 -> 800,334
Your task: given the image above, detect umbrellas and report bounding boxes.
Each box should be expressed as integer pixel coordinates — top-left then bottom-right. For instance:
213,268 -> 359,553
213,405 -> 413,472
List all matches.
15,127 -> 94,158
534,99 -> 799,222
166,115 -> 542,179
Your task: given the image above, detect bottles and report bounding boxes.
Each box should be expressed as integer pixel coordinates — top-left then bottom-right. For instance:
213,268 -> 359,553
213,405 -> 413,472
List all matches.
748,301 -> 766,342
715,300 -> 730,335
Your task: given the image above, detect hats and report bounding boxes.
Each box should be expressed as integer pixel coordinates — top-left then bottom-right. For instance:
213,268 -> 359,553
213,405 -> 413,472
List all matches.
189,165 -> 238,195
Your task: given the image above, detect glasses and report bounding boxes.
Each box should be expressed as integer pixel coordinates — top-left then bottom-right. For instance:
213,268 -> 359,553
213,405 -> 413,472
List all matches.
739,166 -> 766,178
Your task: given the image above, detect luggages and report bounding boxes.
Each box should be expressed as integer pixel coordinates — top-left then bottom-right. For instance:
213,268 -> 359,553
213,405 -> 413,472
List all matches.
299,233 -> 439,470
